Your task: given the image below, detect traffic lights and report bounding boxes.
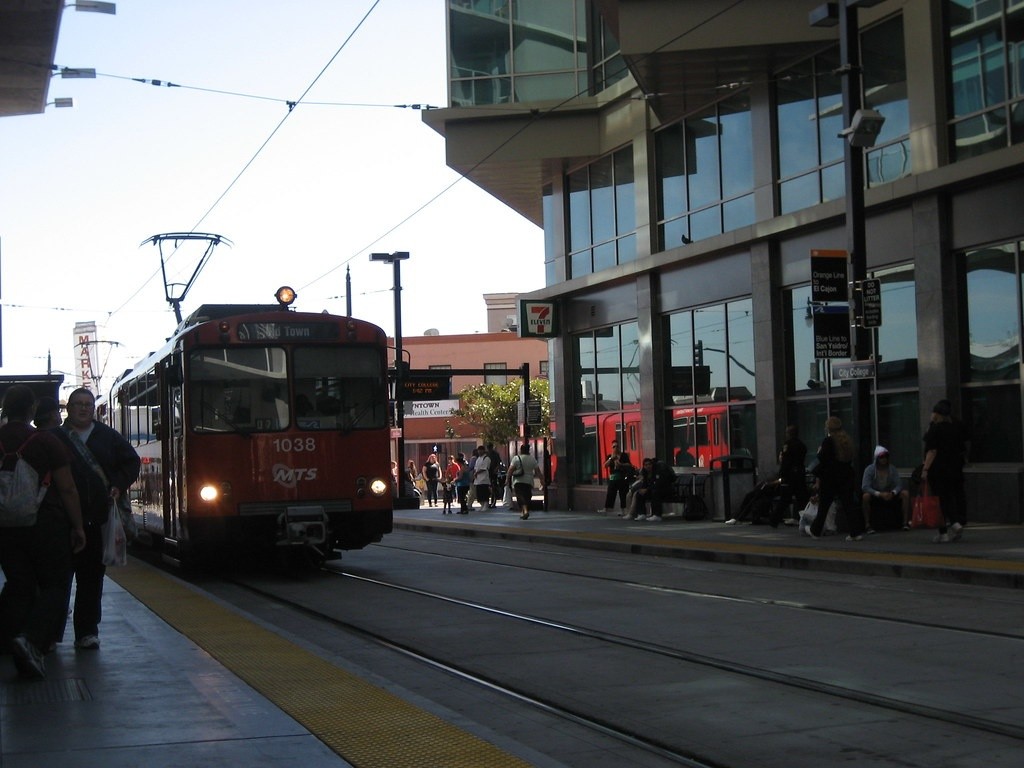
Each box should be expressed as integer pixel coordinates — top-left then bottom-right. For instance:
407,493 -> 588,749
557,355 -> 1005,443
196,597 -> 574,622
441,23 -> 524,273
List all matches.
694,340 -> 703,366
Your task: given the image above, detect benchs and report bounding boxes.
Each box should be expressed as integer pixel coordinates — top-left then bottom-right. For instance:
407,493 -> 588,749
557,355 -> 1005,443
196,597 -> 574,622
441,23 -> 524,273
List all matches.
770,471 -> 819,526
664,474 -> 708,517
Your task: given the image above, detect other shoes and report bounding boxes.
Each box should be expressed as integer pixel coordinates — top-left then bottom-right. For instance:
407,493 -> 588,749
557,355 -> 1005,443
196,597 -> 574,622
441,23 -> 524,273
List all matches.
805,525 -> 820,540
725,518 -> 736,525
901,521 -> 912,531
618,511 -> 625,516
520,513 -> 529,520
12,634 -> 48,679
932,522 -> 963,543
646,515 -> 662,522
845,534 -> 863,541
865,527 -> 876,534
74,633 -> 100,649
622,512 -> 646,521
597,508 -> 607,512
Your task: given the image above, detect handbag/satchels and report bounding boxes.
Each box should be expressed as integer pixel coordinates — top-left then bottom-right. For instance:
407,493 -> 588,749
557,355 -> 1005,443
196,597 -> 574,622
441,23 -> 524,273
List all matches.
511,455 -> 524,477
799,501 -> 818,537
101,497 -> 127,567
909,478 -> 945,528
106,490 -> 138,542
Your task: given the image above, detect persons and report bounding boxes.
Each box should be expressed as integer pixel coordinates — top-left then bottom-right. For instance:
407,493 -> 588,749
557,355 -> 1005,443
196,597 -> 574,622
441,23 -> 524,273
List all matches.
503,444 -> 547,518
0,385 -> 141,676
597,441 -> 676,522
422,442 -> 501,515
404,459 -> 417,488
726,399 -> 970,545
391,460 -> 398,486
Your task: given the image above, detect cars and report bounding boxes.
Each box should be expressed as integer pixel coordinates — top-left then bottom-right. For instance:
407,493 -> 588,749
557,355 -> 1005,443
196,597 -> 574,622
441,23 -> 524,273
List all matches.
498,463 -> 507,496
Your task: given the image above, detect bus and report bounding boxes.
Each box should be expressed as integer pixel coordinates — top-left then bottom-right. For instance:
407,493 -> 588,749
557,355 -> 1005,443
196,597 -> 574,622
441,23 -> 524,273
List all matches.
94,305 -> 395,566
789,354 -> 1024,494
549,386 -> 756,474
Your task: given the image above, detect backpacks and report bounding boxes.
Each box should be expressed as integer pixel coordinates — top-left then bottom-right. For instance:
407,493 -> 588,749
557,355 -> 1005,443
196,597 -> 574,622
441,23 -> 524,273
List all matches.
0,432 -> 52,527
682,495 -> 712,520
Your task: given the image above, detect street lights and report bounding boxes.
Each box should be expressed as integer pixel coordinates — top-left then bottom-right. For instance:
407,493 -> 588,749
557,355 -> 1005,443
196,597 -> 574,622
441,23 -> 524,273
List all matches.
804,301 -> 830,419
371,252 -> 409,504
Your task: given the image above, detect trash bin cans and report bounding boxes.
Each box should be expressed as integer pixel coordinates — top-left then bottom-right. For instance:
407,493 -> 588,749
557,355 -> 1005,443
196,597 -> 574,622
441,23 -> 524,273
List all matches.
709,454 -> 757,522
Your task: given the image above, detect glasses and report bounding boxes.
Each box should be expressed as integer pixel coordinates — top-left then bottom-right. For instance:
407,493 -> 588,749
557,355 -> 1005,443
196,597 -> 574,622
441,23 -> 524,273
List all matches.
56,407 -> 62,412
